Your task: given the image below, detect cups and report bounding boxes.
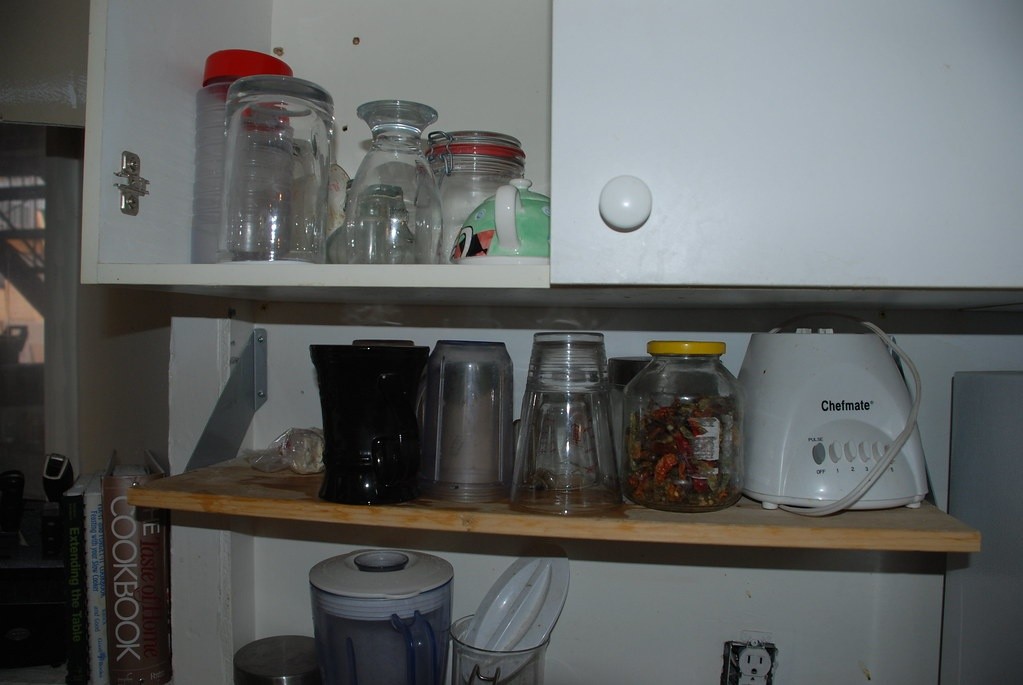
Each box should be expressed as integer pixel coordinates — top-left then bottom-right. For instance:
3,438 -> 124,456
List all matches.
424,130 -> 526,264
215,74 -> 334,264
419,339 -> 514,504
449,615 -> 551,685
309,344 -> 428,505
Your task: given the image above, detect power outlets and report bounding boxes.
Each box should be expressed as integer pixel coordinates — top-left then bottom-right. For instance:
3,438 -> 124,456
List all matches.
719,640 -> 779,685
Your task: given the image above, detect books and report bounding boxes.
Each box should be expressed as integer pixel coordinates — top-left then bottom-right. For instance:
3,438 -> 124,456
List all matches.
62,446 -> 174,685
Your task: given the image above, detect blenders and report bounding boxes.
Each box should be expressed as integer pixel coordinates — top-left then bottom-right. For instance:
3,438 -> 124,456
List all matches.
309,548 -> 455,685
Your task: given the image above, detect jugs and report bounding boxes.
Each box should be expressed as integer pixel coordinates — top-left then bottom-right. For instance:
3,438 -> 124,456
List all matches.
450,178 -> 551,266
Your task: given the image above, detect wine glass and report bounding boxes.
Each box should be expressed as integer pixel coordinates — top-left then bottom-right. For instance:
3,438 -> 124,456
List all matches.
344,99 -> 443,265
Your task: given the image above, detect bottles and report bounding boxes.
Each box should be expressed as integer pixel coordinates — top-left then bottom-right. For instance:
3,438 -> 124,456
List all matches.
621,339 -> 745,513
508,332 -> 622,515
190,49 -> 293,265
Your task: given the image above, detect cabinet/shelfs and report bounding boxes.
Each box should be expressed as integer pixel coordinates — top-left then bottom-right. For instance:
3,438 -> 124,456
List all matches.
79,0 -> 1022,317
